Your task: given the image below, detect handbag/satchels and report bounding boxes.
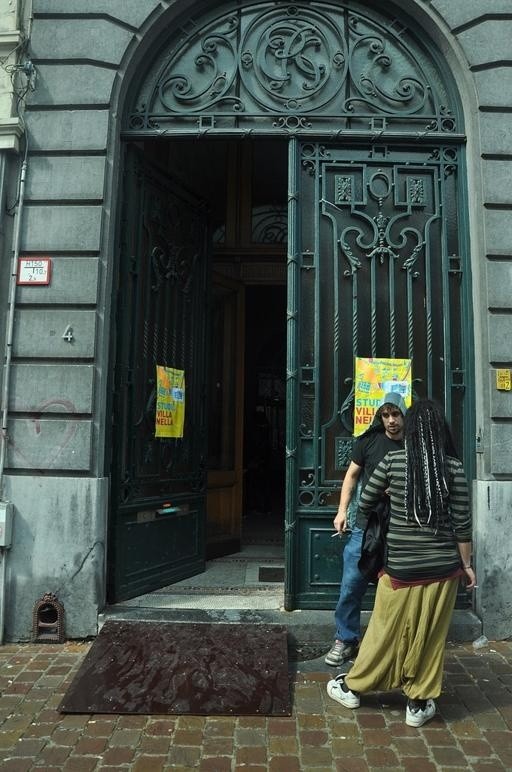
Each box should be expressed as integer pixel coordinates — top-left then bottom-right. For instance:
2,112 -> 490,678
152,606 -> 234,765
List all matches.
358,502 -> 393,573
346,482 -> 364,529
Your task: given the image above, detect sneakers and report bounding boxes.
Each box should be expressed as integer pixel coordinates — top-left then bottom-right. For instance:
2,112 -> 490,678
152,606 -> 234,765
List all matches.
323,635 -> 362,666
405,697 -> 437,728
325,674 -> 361,710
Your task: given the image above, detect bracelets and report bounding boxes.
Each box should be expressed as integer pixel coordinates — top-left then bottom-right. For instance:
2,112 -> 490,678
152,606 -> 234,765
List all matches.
463,565 -> 471,568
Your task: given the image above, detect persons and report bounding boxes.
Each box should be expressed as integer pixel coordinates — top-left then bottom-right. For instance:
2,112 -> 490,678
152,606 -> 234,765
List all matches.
326,400 -> 478,728
325,390 -> 408,669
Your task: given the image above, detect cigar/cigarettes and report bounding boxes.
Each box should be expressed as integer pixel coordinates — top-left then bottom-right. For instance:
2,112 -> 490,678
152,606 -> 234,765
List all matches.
329,529 -> 338,537
